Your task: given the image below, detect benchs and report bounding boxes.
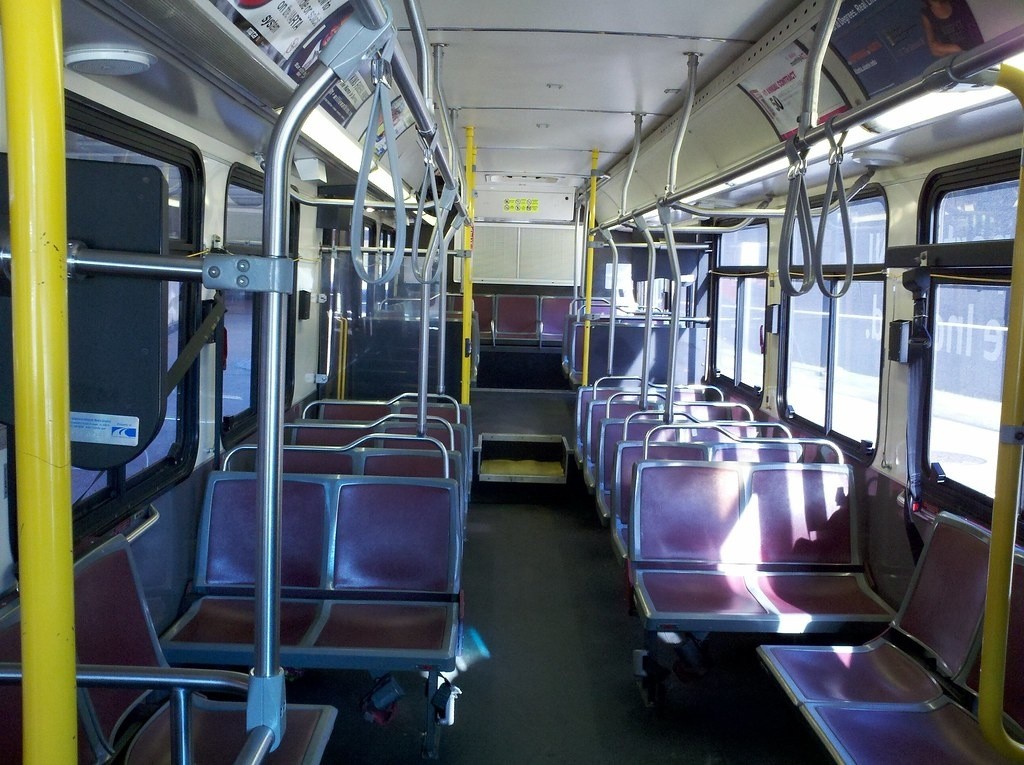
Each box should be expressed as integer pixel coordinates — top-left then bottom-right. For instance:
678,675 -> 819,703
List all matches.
221,444 -> 463,521
630,421 -> 898,711
0,532 -> 340,765
754,510 -> 1024,765
367,285 -> 684,385
583,375 -> 732,487
575,386 -> 707,463
611,409 -> 805,558
595,390 -> 762,517
158,471 -> 463,758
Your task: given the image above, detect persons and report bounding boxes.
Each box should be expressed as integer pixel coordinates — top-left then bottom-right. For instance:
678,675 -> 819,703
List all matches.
920,0 -> 983,59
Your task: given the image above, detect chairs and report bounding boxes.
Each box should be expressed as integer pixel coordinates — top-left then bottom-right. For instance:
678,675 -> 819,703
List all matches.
291,421 -> 467,513
319,403 -> 473,482
